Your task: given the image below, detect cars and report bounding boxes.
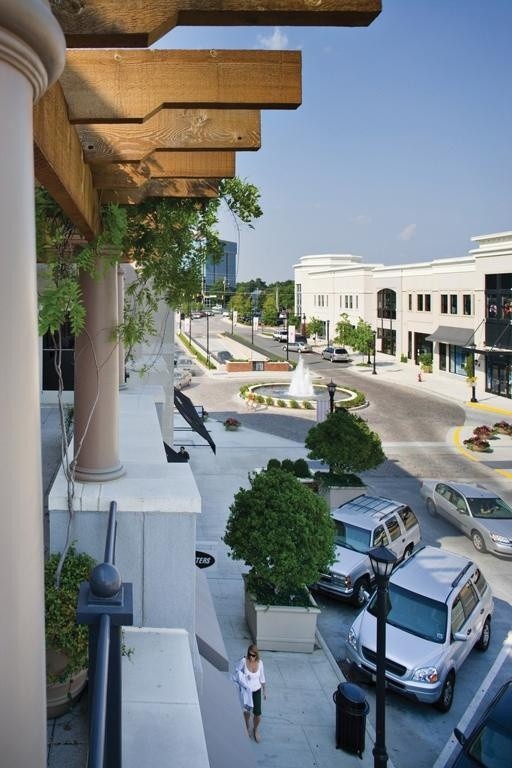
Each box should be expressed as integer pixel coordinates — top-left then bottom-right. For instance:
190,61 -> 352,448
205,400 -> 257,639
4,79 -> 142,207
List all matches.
343,544 -> 495,713
415,477 -> 512,561
300,493 -> 422,610
321,347 -> 350,363
273,329 -> 312,353
450,680 -> 512,768
191,306 -> 232,319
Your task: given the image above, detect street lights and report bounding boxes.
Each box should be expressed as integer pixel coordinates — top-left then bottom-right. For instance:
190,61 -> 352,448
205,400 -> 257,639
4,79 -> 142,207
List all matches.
372,329 -> 377,375
231,303 -> 235,336
367,536 -> 398,768
251,309 -> 254,344
202,304 -> 214,367
285,311 -> 289,360
468,340 -> 478,402
303,314 -> 306,331
188,309 -> 193,346
326,376 -> 337,413
327,320 -> 330,346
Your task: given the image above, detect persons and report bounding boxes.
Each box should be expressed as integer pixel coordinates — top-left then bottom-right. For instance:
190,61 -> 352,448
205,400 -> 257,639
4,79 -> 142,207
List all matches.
480,503 -> 499,514
178,447 -> 189,459
235,645 -> 267,743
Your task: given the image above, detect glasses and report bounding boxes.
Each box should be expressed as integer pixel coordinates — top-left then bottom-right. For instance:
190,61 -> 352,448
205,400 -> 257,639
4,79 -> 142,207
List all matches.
248,652 -> 257,657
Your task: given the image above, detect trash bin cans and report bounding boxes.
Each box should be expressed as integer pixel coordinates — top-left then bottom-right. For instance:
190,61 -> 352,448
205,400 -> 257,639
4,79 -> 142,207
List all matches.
332,681 -> 370,760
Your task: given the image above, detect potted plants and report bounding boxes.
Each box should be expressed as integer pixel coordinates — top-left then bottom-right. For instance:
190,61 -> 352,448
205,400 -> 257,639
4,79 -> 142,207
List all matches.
464,420 -> 512,452
305,407 -> 387,514
222,468 -> 339,652
45,539 -> 135,720
